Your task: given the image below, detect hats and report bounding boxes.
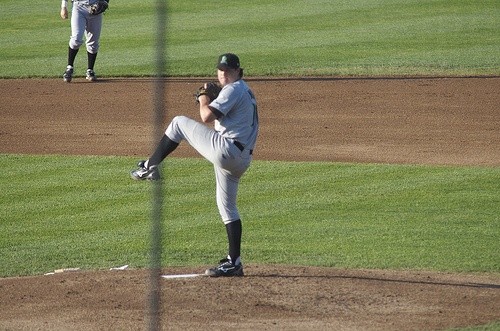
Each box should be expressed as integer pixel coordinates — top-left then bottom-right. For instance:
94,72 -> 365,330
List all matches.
216,53 -> 240,69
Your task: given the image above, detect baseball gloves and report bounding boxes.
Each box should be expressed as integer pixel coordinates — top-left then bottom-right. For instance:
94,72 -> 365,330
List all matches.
195,82 -> 222,103
89,0 -> 109,16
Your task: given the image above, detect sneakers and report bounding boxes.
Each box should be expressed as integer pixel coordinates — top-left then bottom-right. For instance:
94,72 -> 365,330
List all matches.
204,257 -> 244,278
129,159 -> 161,182
62,65 -> 74,83
85,68 -> 95,81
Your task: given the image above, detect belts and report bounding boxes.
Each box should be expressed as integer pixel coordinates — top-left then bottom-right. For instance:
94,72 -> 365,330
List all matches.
232,141 -> 253,156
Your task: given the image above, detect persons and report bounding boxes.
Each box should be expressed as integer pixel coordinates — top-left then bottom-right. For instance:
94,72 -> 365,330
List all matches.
58,0 -> 110,84
130,52 -> 258,278
